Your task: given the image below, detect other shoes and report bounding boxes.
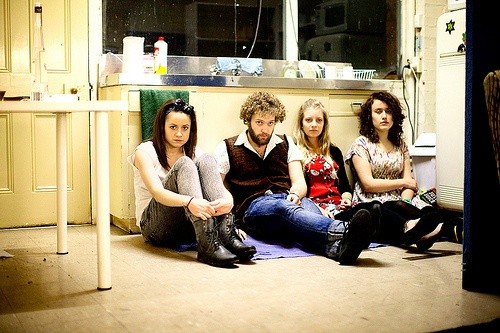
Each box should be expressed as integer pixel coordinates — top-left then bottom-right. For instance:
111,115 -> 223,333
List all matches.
403,215 -> 446,250
335,208 -> 371,265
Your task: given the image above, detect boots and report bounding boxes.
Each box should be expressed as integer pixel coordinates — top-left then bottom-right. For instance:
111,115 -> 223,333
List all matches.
214,212 -> 257,257
193,218 -> 239,266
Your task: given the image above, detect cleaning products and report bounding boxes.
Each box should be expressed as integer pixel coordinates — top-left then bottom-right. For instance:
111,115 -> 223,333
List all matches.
153,36 -> 168,75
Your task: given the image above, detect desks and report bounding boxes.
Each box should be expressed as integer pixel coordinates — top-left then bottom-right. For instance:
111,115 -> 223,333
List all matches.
0,100 -> 127,290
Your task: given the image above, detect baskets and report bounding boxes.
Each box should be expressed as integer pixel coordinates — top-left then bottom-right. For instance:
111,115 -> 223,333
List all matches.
296,69 -> 376,79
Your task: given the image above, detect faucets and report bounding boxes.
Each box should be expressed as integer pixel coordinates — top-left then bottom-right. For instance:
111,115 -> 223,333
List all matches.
232,59 -> 240,76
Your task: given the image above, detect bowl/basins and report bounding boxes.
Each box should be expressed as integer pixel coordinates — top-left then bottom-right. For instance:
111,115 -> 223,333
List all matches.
285,60 -> 327,79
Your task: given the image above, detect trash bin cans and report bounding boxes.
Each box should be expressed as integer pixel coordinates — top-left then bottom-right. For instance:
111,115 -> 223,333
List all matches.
409,133 -> 436,192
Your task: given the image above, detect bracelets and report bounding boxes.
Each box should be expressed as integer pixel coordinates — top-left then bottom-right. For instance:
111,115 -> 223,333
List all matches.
342,197 -> 351,201
290,192 -> 299,198
187,197 -> 195,207
402,198 -> 413,204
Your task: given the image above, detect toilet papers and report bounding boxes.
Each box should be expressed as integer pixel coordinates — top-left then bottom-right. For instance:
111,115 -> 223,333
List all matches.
122,36 -> 145,74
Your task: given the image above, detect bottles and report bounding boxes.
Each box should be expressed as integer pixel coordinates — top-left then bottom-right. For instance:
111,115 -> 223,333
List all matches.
154,36 -> 168,74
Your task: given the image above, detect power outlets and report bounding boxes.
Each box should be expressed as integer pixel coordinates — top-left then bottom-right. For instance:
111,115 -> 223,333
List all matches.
411,57 -> 422,74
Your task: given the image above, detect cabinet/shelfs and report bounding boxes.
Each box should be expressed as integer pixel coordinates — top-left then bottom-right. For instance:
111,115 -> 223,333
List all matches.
185,2 -> 281,59
99,85 -> 388,234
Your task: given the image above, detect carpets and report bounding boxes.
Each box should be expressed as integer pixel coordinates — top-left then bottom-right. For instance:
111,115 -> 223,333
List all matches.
173,229 -> 390,260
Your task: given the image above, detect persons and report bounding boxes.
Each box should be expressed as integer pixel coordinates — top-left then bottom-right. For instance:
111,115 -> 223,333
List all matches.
126,98 -> 256,264
217,91 -> 373,265
293,99 -> 445,250
345,90 -> 464,243
